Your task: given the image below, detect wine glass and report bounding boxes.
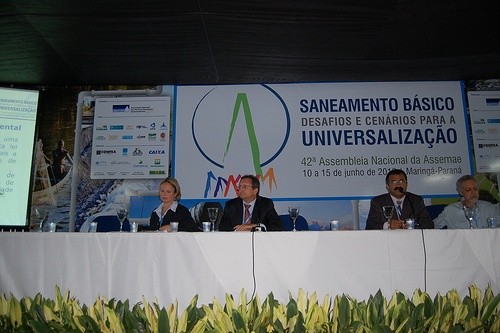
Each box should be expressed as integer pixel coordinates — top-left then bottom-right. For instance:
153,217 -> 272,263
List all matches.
382,206 -> 396,230
463,205 -> 476,229
206,207 -> 219,232
115,208 -> 128,232
34,208 -> 49,232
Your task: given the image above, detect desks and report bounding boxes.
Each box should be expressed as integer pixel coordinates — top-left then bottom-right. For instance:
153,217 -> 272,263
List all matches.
0,229 -> 500,313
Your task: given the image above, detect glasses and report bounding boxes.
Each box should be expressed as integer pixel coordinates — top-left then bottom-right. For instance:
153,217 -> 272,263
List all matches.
388,180 -> 407,184
236,184 -> 254,188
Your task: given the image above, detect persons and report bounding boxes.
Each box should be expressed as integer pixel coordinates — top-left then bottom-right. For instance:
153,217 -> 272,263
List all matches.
150,177 -> 197,232
365,169 -> 434,230
434,175 -> 500,229
52,139 -> 73,176
218,175 -> 282,231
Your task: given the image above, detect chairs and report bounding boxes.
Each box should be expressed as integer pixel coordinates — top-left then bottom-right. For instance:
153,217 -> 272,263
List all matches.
88,215 -> 130,233
425,204 -> 449,229
280,214 -> 309,231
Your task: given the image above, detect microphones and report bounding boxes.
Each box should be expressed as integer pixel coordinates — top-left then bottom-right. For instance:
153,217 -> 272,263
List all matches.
399,188 -> 415,219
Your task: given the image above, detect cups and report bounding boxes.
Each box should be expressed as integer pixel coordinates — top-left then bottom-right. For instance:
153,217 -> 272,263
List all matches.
488,217 -> 497,228
47,222 -> 56,233
169,222 -> 179,232
130,221 -> 138,233
89,223 -> 97,232
202,222 -> 212,232
329,219 -> 341,230
405,218 -> 415,230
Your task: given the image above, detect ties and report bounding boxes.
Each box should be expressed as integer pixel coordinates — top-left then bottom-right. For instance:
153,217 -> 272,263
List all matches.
396,200 -> 402,216
243,203 -> 251,223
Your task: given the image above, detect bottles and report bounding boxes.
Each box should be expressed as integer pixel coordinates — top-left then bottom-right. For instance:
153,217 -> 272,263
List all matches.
288,206 -> 301,231
